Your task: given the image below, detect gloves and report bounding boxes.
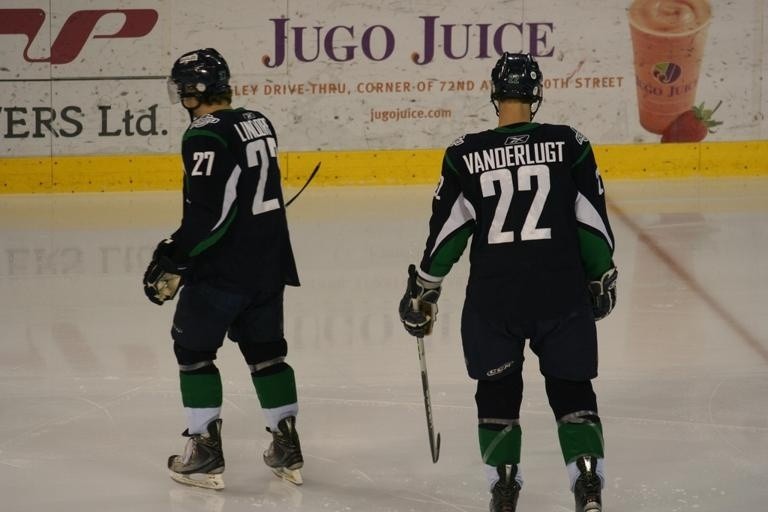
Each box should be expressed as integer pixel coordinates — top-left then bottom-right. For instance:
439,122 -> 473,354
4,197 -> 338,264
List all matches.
143,237 -> 195,305
398,263 -> 440,338
589,266 -> 618,321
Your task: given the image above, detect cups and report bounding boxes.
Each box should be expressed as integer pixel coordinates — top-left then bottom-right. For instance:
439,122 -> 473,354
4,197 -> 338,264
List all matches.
626,0 -> 715,135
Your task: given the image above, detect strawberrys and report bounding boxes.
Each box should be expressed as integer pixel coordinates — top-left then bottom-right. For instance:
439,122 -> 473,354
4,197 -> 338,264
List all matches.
660,98 -> 724,143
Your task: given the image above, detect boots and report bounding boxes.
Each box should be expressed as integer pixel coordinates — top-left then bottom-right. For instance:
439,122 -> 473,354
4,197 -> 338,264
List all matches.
264,415 -> 304,470
574,456 -> 602,512
490,463 -> 520,512
168,417 -> 226,474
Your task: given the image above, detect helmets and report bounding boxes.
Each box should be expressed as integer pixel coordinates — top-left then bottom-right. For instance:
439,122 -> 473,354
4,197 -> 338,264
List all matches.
167,48 -> 230,122
491,52 -> 542,122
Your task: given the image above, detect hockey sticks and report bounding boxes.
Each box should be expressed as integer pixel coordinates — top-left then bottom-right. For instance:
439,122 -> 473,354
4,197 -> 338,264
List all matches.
405,263 -> 442,464
147,161 -> 323,292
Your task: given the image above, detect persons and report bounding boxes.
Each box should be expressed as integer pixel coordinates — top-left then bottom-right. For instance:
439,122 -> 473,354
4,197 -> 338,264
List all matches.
142,48 -> 303,474
398,51 -> 619,512
